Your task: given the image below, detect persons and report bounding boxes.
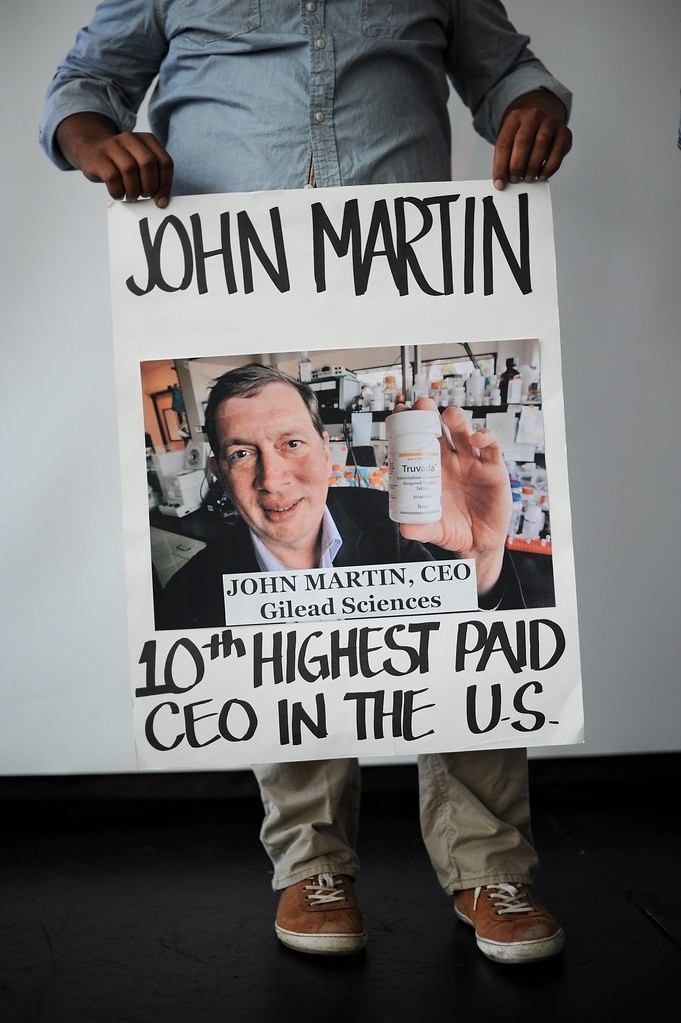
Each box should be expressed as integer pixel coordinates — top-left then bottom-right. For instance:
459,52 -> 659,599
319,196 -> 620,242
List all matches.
161,363 -> 554,627
38,0 -> 574,964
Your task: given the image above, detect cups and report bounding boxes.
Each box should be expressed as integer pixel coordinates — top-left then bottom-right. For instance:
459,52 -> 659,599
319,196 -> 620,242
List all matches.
351,413 -> 372,446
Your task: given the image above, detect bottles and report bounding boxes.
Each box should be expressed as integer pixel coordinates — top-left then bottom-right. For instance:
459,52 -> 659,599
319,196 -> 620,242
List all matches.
351,357 -> 540,411
298,351 -> 312,382
326,411 -> 442,524
507,473 -> 550,546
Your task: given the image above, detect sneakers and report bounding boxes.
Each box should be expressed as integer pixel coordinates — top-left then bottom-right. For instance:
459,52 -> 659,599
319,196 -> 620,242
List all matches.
454,883 -> 565,963
274,873 -> 367,954
